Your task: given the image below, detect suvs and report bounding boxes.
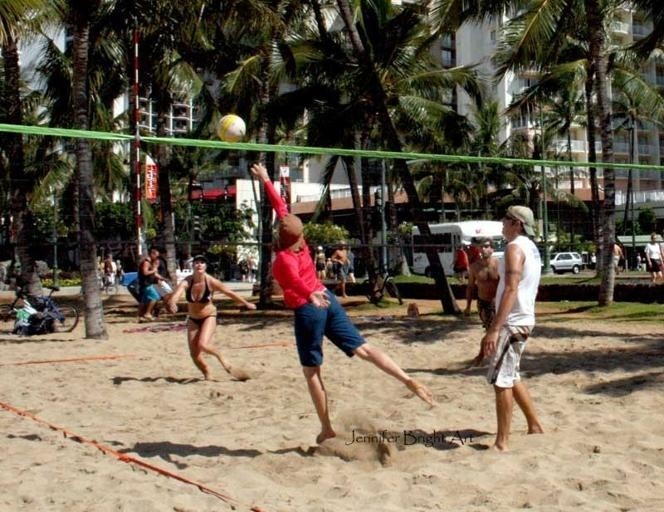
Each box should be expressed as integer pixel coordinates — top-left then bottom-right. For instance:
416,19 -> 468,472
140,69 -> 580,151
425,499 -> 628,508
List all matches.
540,251 -> 582,274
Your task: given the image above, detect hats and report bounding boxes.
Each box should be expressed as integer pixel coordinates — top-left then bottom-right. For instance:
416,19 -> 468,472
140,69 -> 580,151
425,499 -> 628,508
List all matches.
317,245 -> 323,250
506,205 -> 535,236
336,240 -> 348,245
279,214 -> 303,251
194,255 -> 206,262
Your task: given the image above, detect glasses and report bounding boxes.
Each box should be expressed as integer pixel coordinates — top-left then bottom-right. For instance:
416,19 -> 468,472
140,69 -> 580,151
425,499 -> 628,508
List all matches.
480,244 -> 491,248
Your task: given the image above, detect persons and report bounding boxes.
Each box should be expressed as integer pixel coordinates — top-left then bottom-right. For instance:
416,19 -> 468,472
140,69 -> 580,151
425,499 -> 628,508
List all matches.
462,237 -> 501,368
10,295 -> 38,336
479,204 -> 545,452
308,239 -> 356,299
166,254 -> 256,382
590,231 -> 664,283
92,243 -> 261,322
248,162 -> 433,446
455,235 -> 483,284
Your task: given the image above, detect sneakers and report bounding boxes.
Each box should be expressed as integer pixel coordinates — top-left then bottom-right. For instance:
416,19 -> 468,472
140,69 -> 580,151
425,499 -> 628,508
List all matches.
140,314 -> 157,321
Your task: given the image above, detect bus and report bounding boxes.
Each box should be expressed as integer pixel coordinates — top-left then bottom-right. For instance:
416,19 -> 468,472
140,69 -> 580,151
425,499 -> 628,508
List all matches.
412,218 -> 503,277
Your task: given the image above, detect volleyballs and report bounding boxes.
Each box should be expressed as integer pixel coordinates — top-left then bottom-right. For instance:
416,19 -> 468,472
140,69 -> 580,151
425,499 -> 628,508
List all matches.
215,114 -> 246,143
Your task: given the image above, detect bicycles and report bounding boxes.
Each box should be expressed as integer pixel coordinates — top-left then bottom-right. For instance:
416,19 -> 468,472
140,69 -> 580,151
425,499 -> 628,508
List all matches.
0,273 -> 79,333
362,262 -> 402,307
